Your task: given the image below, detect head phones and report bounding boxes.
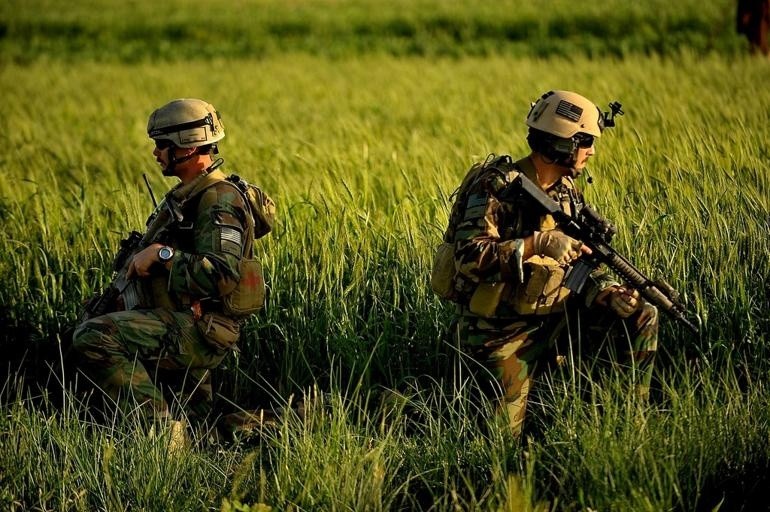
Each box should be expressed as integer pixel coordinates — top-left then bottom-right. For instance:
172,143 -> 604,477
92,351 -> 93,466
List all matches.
543,133 -> 579,167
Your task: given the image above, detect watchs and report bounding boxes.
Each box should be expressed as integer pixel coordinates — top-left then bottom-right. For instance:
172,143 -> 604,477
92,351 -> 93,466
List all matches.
159,245 -> 173,265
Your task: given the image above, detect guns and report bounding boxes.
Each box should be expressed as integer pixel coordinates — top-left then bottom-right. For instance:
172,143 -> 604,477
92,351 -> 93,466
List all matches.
66,193 -> 184,336
501,174 -> 699,335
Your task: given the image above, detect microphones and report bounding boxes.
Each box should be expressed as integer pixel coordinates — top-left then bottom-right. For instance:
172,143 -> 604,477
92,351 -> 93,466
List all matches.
583,167 -> 593,183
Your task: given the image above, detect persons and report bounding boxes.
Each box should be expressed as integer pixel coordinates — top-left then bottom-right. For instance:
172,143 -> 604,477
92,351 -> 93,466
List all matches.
454,89 -> 660,452
72,99 -> 257,460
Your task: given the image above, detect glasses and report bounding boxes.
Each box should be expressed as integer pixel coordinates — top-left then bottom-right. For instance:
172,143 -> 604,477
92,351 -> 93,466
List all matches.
154,140 -> 173,149
578,138 -> 594,148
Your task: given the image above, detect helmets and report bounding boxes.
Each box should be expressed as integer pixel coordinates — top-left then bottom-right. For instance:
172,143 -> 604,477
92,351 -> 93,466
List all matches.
147,99 -> 225,150
525,90 -> 603,139
